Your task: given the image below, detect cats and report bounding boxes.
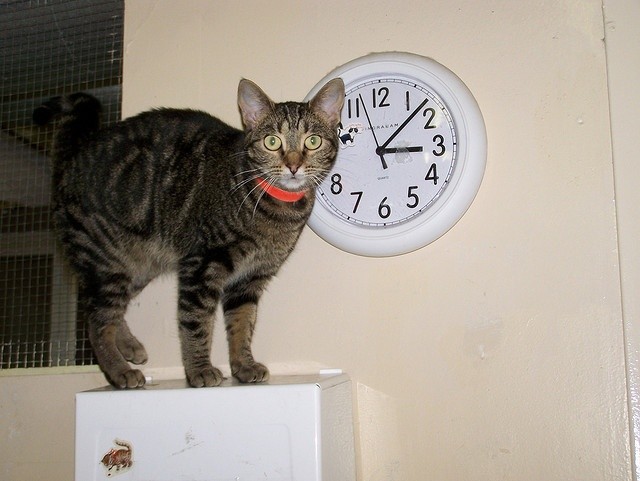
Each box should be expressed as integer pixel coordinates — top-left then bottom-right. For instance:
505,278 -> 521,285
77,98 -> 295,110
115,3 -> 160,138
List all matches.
32,77 -> 345,388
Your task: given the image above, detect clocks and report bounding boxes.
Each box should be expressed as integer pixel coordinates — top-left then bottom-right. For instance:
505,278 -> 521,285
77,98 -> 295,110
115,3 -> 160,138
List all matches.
302,51 -> 487,257
